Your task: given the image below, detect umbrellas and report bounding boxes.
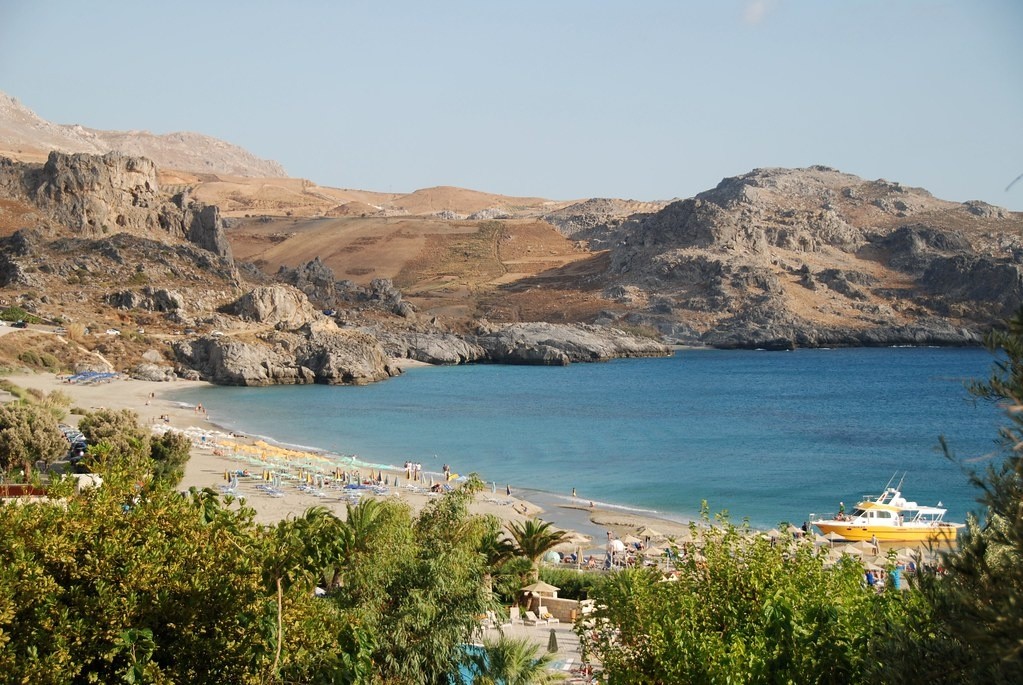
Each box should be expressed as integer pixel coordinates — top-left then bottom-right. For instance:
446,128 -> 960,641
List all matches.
547,629 -> 558,653
153,422 -> 512,501
542,520 -> 925,584
520,580 -> 561,620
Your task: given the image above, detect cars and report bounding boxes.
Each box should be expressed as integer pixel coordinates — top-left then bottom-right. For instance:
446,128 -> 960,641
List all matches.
57,423 -> 87,459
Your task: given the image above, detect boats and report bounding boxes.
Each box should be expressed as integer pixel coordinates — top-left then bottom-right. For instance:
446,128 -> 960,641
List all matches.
809,469 -> 967,543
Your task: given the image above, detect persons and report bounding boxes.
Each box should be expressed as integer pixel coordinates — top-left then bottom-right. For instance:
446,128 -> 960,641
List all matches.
871,534 -> 879,556
590,501 -> 594,507
873,569 -> 879,581
837,501 -> 854,522
866,570 -> 874,588
572,487 -> 577,496
802,521 -> 808,538
880,570 -> 886,580
899,514 -> 905,527
